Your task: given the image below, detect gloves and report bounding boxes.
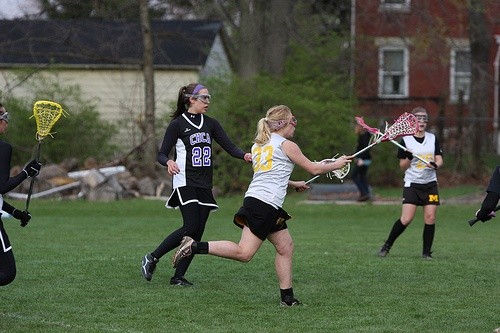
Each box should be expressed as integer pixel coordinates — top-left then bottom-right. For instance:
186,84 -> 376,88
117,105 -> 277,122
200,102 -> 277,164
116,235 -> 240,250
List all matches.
406,150 -> 414,160
12,208 -> 31,227
24,160 -> 42,178
429,161 -> 437,169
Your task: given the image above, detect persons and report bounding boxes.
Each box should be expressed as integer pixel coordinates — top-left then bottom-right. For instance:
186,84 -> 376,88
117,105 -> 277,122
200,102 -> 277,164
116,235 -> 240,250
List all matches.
349,116 -> 374,202
0,104 -> 42,288
375,107 -> 446,261
141,83 -> 254,288
476,157 -> 500,223
171,103 -> 353,310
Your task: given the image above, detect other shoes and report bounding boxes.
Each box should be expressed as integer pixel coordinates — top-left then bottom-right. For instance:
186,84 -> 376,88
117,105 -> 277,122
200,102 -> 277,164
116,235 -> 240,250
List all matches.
358,195 -> 370,201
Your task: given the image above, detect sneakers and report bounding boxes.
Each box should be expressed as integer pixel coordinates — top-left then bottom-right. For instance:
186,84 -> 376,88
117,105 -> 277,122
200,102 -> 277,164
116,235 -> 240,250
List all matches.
422,251 -> 434,262
280,300 -> 307,308
170,275 -> 194,287
379,241 -> 391,258
141,253 -> 159,282
173,235 -> 195,268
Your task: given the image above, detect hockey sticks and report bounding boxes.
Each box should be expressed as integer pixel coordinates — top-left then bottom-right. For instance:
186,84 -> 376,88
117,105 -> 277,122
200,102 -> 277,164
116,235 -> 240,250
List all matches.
311,153 -> 350,180
295,112 -> 419,191
20,101 -> 70,227
467,205 -> 500,227
355,116 -> 439,172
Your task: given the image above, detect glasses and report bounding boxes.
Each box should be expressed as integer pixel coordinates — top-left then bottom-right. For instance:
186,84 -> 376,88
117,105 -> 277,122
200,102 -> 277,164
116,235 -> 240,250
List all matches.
412,114 -> 429,123
0,112 -> 9,123
184,93 -> 212,103
288,116 -> 298,125
